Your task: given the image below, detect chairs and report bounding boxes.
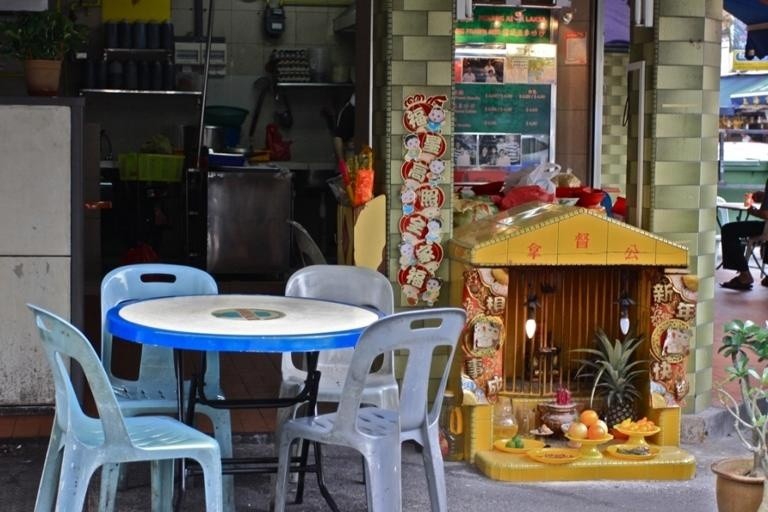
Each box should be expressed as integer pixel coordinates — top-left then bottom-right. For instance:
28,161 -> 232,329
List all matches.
736,214 -> 768,282
716,194 -> 731,266
92,263 -> 241,512
15,298 -> 229,512
273,301 -> 471,511
265,264 -> 406,512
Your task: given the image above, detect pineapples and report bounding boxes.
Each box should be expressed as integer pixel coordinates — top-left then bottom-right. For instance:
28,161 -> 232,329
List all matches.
599,401 -> 639,428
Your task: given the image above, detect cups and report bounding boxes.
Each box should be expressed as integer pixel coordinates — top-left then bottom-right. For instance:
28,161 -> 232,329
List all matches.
331,63 -> 350,82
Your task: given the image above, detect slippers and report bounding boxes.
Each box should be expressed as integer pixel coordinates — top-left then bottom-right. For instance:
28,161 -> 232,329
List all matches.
720,276 -> 753,290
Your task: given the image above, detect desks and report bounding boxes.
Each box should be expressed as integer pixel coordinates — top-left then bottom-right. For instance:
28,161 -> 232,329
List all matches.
101,289 -> 389,512
716,201 -> 766,281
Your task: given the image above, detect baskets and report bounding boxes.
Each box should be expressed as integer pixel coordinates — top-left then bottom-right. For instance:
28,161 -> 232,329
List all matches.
117,153 -> 185,183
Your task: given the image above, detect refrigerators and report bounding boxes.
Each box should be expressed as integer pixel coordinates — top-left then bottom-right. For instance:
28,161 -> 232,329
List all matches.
0,102 -> 71,410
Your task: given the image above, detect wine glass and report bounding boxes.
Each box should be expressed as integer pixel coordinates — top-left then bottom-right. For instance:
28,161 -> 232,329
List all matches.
563,430 -> 614,460
613,423 -> 661,450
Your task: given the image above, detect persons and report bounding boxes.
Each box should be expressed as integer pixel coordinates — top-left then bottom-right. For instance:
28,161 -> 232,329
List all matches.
462,59 -> 498,82
719,178 -> 768,290
454,135 -> 520,167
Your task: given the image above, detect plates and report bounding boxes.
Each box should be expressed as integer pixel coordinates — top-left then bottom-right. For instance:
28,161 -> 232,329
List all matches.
605,444 -> 662,461
492,439 -> 545,454
524,448 -> 582,465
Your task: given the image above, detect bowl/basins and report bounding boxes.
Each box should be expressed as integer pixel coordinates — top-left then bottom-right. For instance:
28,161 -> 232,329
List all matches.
203,104 -> 249,126
556,187 -> 605,207
470,180 -> 504,196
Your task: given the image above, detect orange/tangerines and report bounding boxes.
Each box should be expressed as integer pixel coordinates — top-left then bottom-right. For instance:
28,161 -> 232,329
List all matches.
505,434 -> 523,448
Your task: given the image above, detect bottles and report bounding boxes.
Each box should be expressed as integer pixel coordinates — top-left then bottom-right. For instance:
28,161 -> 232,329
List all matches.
307,47 -> 322,83
94,16 -> 177,89
493,399 -> 518,441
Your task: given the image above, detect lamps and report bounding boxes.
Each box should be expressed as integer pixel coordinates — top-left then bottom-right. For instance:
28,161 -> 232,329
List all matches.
610,288 -> 639,337
522,293 -> 537,340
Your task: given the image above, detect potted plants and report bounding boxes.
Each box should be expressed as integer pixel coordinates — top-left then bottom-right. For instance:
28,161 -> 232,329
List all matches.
699,309 -> 767,512
0,7 -> 96,96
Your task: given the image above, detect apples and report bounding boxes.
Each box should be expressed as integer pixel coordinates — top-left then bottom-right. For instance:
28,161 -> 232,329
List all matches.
568,409 -> 608,439
